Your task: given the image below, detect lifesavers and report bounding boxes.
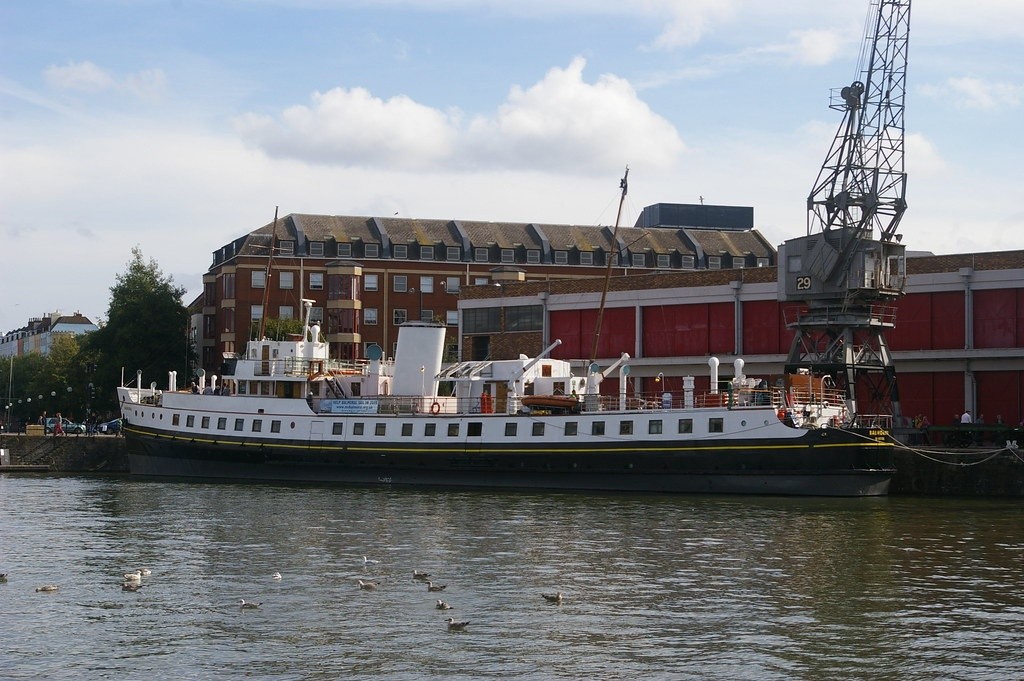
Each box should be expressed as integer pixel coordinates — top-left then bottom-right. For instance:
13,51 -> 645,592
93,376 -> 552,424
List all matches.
431,402 -> 440,415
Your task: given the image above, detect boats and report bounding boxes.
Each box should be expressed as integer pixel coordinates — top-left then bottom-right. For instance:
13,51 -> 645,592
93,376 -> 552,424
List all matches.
118,165 -> 897,498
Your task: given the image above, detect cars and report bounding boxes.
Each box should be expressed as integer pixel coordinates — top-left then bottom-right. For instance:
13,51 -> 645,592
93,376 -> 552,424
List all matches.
46,418 -> 87,434
100,419 -> 122,435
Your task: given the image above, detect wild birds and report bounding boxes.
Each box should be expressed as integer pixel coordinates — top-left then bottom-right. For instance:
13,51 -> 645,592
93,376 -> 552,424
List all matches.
123,568 -> 151,580
411,568 -> 472,630
538,591 -> 563,603
362,555 -> 380,565
33,582 -> 61,593
357,579 -> 377,590
272,571 -> 282,580
235,598 -> 260,609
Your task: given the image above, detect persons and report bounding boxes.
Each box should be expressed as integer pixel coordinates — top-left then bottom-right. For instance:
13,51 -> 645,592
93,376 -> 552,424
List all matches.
38,410 -> 47,436
975,414 -> 984,423
86,412 -> 99,436
953,410 -> 973,424
995,415 -> 1005,424
203,383 -> 213,394
214,383 -> 230,396
54,413 -> 64,436
570,390 -> 578,399
191,382 -> 201,394
912,413 -> 929,428
